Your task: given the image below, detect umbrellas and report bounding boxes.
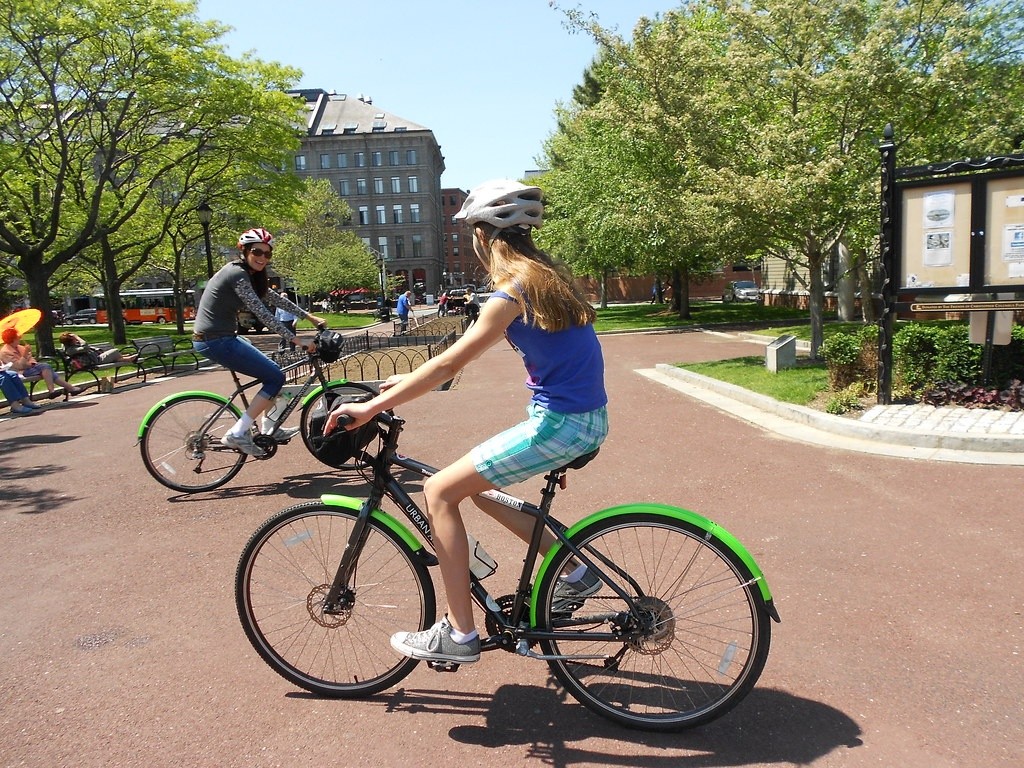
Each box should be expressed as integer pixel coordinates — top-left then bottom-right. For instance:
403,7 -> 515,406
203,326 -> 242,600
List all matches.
0,309 -> 41,346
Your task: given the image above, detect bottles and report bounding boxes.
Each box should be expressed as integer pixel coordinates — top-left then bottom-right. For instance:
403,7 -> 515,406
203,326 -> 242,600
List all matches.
465,533 -> 496,580
267,392 -> 293,421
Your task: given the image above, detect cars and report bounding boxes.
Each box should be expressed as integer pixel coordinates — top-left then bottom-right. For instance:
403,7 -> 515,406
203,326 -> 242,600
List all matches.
721,281 -> 762,303
449,284 -> 495,314
235,299 -> 277,335
63,309 -> 98,324
53,310 -> 66,325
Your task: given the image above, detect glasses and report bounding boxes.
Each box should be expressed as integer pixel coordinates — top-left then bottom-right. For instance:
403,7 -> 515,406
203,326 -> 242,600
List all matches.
249,249 -> 273,259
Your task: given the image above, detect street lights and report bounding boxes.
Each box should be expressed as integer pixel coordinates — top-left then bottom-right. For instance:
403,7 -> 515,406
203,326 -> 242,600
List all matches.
195,202 -> 215,280
376,258 -> 386,307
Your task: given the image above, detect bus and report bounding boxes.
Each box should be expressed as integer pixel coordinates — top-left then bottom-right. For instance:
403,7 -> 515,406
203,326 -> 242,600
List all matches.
93,288 -> 198,325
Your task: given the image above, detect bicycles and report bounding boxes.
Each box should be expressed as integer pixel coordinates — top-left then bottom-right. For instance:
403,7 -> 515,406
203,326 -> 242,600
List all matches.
232,392 -> 782,734
134,322 -> 395,495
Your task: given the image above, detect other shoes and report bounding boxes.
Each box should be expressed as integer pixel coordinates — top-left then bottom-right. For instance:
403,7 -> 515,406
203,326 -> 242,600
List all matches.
129,353 -> 139,361
11,407 -> 32,414
23,403 -> 41,408
48,390 -> 62,400
71,386 -> 87,395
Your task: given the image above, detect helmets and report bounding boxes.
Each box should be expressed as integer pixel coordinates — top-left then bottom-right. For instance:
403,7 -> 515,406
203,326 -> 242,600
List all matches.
454,179 -> 544,228
238,227 -> 274,251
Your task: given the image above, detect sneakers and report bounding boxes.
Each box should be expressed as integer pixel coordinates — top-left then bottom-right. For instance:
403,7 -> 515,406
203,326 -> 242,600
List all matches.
391,614 -> 481,663
270,427 -> 301,440
551,564 -> 603,610
220,430 -> 264,456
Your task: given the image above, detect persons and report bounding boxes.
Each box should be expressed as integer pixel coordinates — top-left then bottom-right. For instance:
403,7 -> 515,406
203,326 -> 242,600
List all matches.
322,297 -> 332,313
437,292 -> 453,318
650,278 -> 671,304
59,333 -> 138,363
321,181 -> 608,661
397,291 -> 411,336
0,329 -> 89,400
275,293 -> 298,359
192,228 -> 328,456
0,360 -> 41,413
384,297 -> 392,315
464,288 -> 479,323
377,294 -> 382,311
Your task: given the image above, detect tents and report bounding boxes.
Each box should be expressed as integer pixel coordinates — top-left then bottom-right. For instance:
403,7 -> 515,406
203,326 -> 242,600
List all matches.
330,289 -> 382,294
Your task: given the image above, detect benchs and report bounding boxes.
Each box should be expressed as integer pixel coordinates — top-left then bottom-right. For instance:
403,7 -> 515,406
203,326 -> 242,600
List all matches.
21,334 -> 198,402
